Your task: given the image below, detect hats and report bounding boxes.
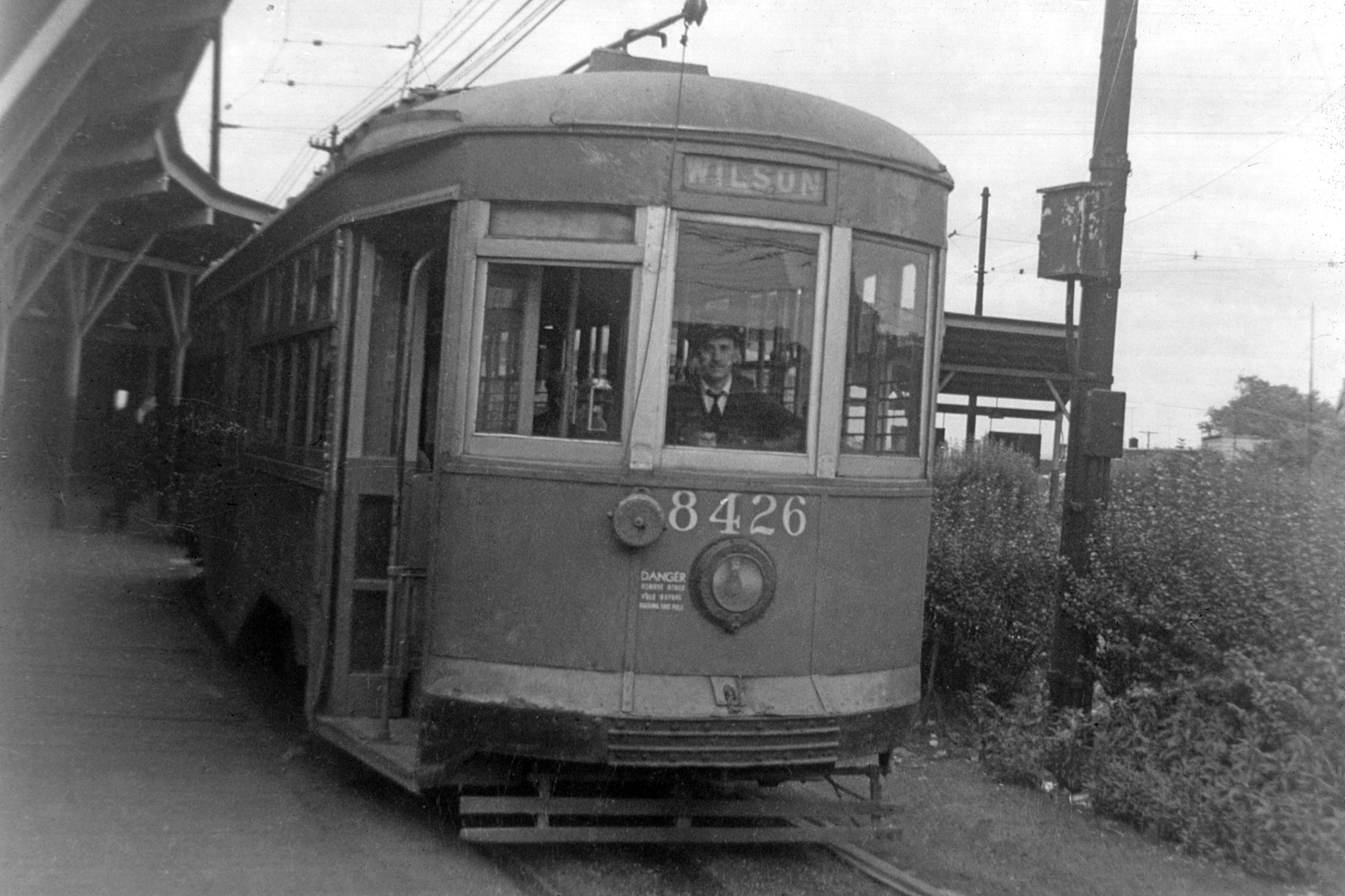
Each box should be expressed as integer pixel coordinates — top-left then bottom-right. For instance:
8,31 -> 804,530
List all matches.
689,325 -> 745,346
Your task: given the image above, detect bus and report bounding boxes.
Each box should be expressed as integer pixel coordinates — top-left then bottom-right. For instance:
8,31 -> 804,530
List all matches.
176,0 -> 960,838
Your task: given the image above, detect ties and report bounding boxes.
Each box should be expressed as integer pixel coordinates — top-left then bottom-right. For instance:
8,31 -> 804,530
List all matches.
705,389 -> 727,426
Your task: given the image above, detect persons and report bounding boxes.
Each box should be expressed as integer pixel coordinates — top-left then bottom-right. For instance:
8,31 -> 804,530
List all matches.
667,308 -> 808,452
95,384 -> 171,533
